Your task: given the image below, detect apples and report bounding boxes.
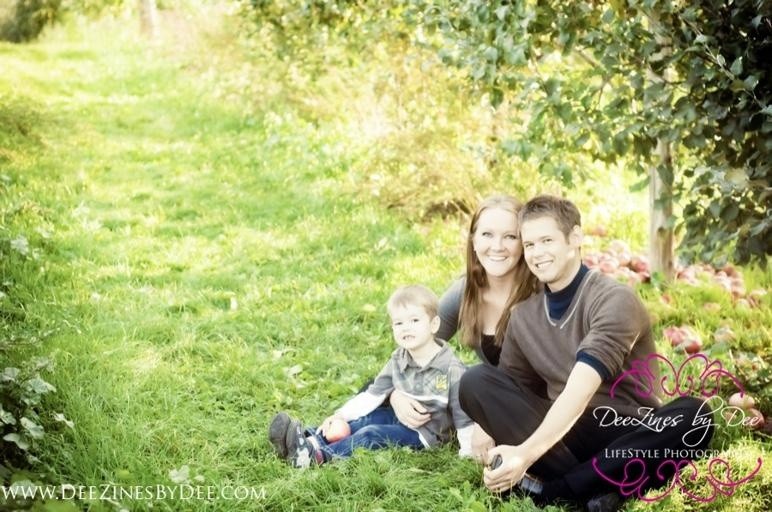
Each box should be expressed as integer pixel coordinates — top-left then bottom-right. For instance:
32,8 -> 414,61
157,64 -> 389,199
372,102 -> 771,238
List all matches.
584,224 -> 772,432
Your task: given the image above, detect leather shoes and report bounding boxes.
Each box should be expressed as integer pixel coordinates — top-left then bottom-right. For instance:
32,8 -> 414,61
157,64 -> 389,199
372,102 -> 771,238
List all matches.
488,455 -> 551,509
573,489 -> 627,512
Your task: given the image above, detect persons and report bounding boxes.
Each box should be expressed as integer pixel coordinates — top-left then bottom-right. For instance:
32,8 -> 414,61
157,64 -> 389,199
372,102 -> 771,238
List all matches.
456,195 -> 715,511
266,282 -> 474,470
388,193 -> 543,428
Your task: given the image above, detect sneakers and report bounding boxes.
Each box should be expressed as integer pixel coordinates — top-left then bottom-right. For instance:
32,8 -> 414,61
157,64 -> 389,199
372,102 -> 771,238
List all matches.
286,420 -> 318,470
268,414 -> 292,463
